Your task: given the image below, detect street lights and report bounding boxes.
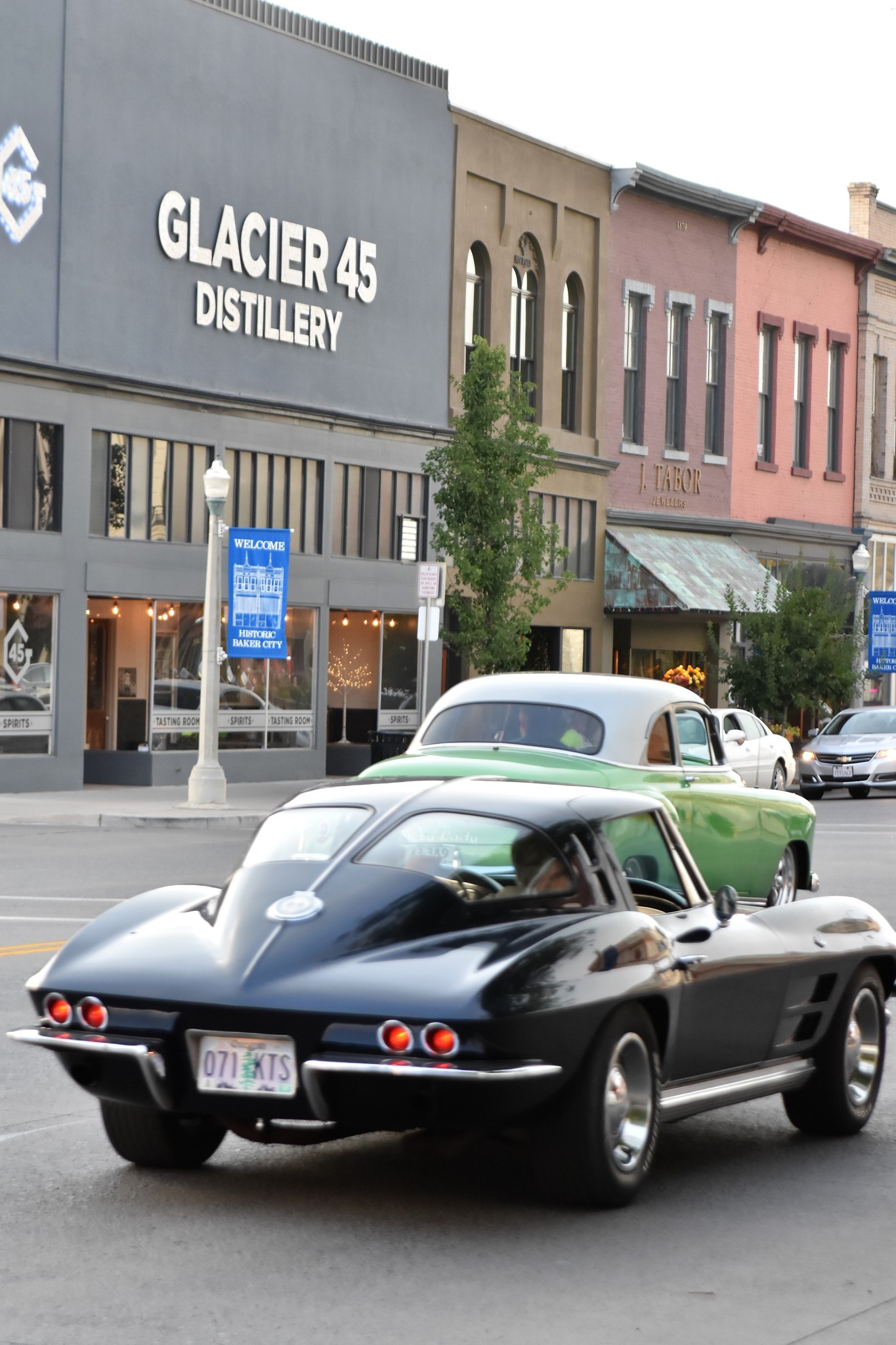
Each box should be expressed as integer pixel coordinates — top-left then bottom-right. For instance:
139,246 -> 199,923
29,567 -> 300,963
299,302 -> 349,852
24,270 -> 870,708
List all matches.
851,541 -> 873,707
184,454 -> 231,807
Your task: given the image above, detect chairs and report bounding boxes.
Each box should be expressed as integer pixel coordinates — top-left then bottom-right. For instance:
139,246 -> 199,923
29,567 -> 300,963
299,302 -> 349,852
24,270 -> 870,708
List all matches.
508,828 -> 579,897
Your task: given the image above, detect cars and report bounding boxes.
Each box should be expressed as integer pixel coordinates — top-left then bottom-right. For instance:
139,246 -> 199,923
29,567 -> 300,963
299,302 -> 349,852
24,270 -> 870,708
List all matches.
798,704 -> 896,801
676,710 -> 799,791
346,671 -> 820,906
0,693 -> 49,754
8,779 -> 895,1212
152,678 -> 311,747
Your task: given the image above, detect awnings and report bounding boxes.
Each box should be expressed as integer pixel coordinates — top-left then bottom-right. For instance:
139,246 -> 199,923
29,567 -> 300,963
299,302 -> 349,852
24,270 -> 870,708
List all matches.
599,527 -> 793,621
